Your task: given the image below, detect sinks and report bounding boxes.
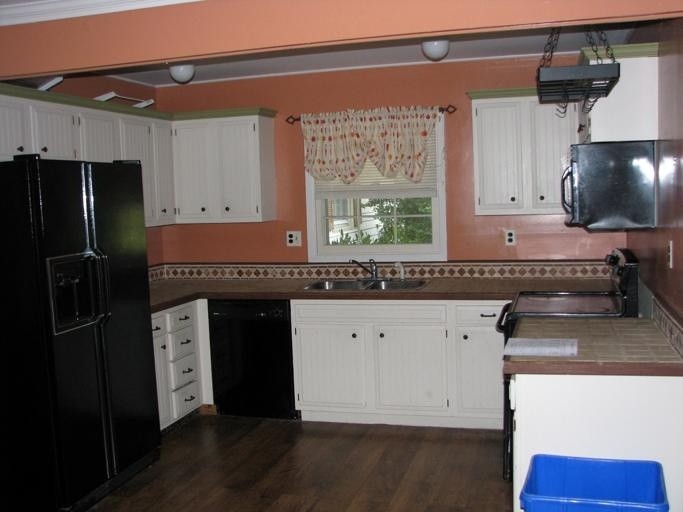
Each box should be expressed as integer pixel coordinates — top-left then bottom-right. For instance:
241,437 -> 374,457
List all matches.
365,278 -> 431,292
295,279 -> 367,291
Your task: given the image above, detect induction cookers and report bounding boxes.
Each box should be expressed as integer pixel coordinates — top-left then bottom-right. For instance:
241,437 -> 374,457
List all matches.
510,291 -> 622,318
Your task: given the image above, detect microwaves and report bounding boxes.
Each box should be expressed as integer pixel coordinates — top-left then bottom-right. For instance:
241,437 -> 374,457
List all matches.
561,142 -> 657,232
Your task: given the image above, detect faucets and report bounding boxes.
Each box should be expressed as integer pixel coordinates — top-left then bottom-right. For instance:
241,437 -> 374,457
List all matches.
394,263 -> 406,281
349,259 -> 378,278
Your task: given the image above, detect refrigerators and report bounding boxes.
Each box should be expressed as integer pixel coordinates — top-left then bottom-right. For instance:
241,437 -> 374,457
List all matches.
0,155 -> 156,511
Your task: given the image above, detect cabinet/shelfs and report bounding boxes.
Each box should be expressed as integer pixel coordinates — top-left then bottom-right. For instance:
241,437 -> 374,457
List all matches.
453,305 -> 505,410
294,303 -> 450,406
0,101 -> 76,161
152,315 -> 172,428
173,121 -> 258,219
78,114 -> 153,221
154,122 -> 172,219
475,99 -> 575,211
165,305 -> 200,420
